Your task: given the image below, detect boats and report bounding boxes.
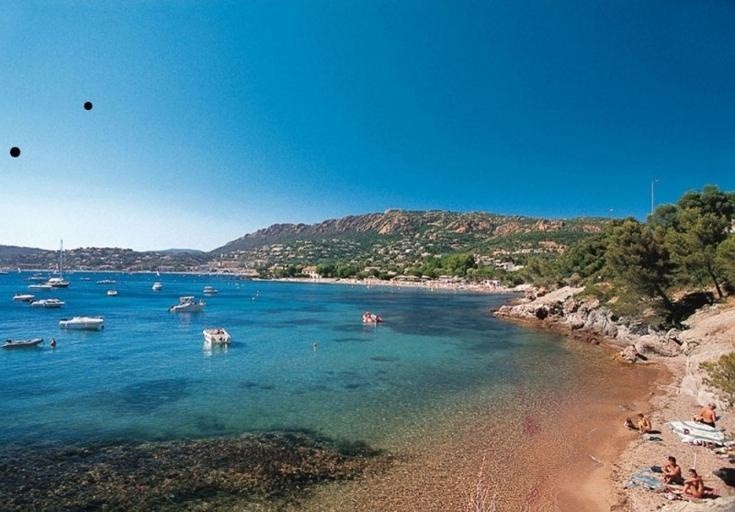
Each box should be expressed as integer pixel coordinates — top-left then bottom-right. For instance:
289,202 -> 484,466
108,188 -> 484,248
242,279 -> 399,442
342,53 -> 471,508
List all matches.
170,295 -> 207,314
202,286 -> 218,296
202,327 -> 230,346
0,268 -> 163,309
2,336 -> 42,349
361,312 -> 383,323
59,316 -> 105,330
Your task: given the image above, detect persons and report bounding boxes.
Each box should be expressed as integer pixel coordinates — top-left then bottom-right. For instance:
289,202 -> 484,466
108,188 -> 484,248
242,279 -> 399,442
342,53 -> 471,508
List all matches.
682,469 -> 704,498
622,417 -> 637,431
694,403 -> 717,426
660,456 -> 682,485
637,414 -> 652,434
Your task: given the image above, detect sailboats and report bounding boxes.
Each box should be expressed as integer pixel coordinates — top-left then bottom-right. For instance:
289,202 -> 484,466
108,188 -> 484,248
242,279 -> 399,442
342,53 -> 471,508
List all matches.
49,240 -> 70,287
